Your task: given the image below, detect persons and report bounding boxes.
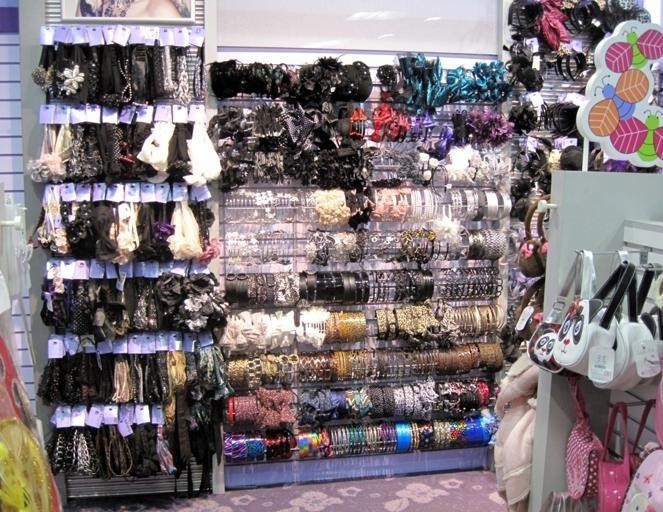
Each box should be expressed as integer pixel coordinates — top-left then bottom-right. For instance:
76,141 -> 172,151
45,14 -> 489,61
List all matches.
74,0 -> 181,19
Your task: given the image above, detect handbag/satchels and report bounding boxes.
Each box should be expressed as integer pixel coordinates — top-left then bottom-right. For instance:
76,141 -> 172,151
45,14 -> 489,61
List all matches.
528,298 -> 662,391
565,422 -> 663,511
